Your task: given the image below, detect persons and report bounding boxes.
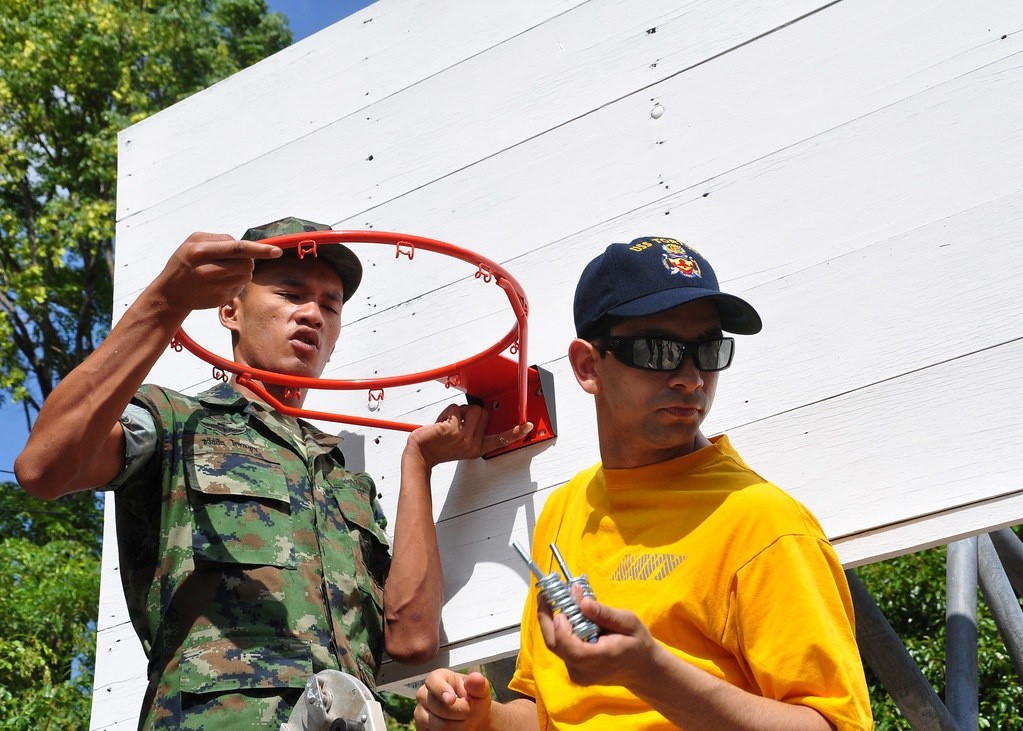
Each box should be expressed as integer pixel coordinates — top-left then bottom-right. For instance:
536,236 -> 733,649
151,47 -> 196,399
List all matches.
414,236 -> 875,731
15,216 -> 532,731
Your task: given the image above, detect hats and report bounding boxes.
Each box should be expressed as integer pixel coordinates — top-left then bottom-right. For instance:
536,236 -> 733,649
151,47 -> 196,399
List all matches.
240,217 -> 362,305
573,237 -> 762,339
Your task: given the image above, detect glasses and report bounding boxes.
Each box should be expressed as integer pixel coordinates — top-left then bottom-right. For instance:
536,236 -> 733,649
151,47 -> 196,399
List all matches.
591,336 -> 736,373
